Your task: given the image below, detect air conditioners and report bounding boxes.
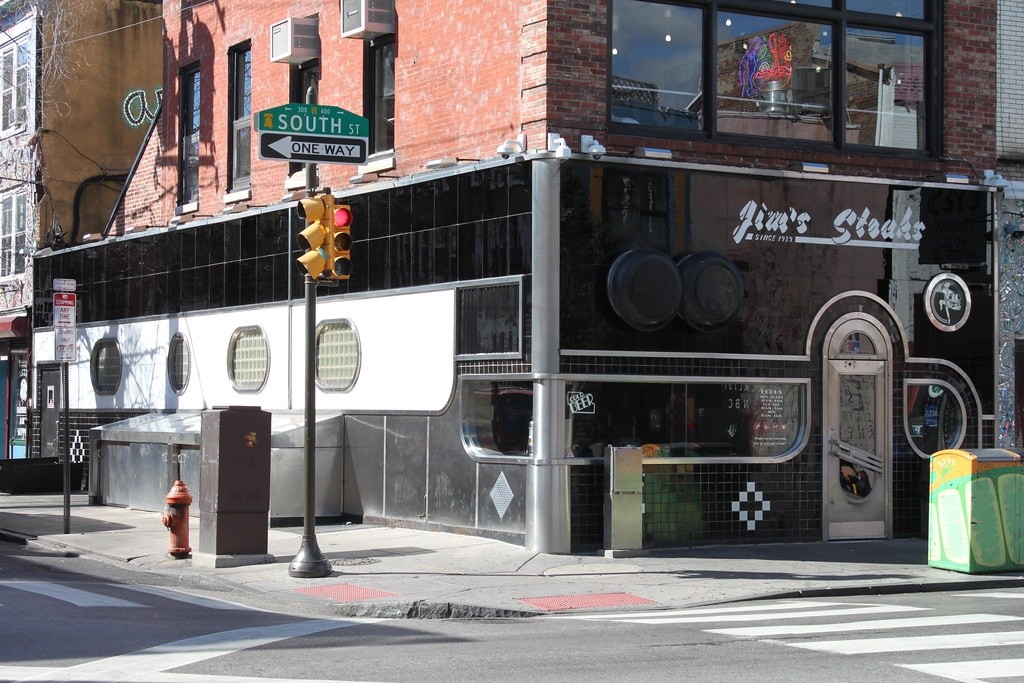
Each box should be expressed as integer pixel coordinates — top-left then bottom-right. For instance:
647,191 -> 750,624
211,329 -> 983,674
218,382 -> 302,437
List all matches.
270,17 -> 319,65
340,0 -> 396,40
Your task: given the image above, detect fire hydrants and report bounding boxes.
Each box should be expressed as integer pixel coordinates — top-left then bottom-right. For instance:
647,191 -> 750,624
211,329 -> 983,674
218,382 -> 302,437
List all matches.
162,480 -> 192,559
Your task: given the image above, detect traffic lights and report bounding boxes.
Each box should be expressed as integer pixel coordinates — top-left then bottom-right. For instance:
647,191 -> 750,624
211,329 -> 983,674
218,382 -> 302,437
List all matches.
296,194 -> 334,279
329,205 -> 352,279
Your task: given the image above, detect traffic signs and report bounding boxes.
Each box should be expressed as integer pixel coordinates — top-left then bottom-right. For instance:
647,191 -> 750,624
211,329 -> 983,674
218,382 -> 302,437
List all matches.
258,103 -> 370,137
259,133 -> 370,166
53,293 -> 76,327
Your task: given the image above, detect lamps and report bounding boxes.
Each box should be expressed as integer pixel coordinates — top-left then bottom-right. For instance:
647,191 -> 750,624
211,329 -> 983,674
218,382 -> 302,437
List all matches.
126,224 -> 168,233
801,162 -> 829,173
350,173 -> 400,184
282,191 -> 307,202
223,205 -> 268,215
171,214 -> 213,224
944,174 -> 969,184
83,232 -> 122,243
426,157 -> 481,169
36,247 -> 68,258
639,148 -> 671,159
